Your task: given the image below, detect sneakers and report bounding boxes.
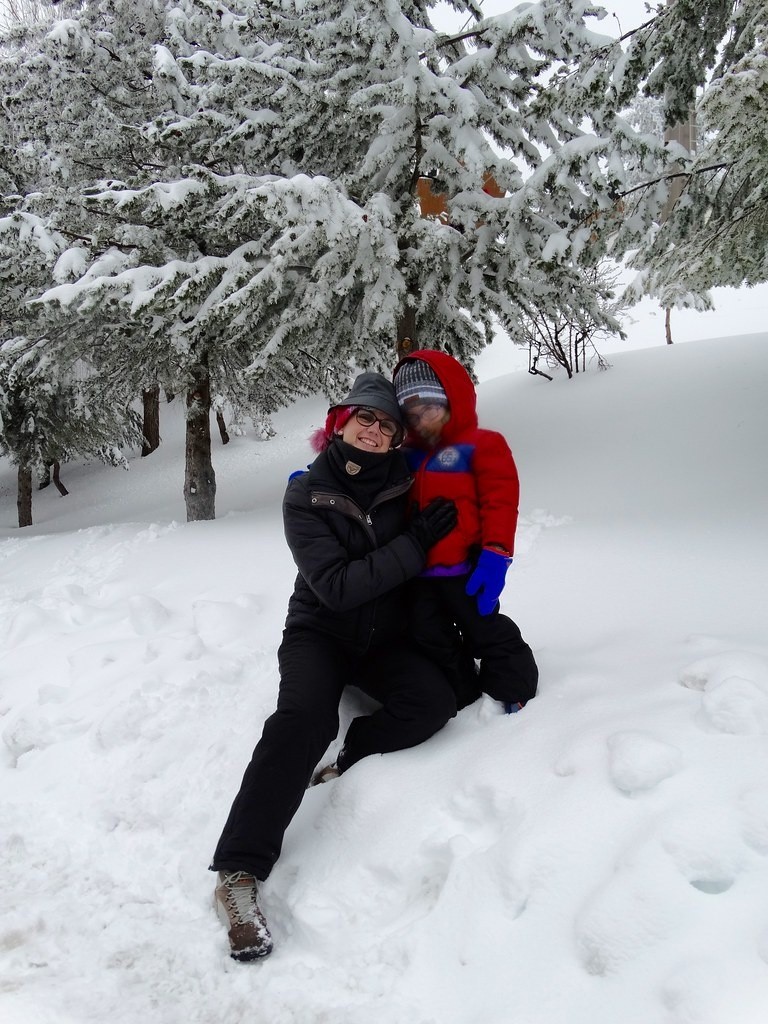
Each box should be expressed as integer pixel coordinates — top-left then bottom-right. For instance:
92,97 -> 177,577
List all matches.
211,870 -> 273,961
307,762 -> 344,789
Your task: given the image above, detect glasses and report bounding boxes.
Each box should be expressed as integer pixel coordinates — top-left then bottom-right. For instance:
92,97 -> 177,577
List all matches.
356,408 -> 398,437
404,404 -> 444,429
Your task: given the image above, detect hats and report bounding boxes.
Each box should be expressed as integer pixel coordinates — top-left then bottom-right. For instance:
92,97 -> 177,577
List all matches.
309,406 -> 358,453
328,372 -> 407,448
393,360 -> 447,412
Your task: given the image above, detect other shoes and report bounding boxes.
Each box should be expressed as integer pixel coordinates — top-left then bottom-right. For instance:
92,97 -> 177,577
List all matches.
505,700 -> 527,713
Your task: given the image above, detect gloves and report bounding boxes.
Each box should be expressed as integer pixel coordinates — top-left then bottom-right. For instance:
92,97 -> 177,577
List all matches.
406,496 -> 458,551
465,546 -> 513,616
288,463 -> 313,483
466,544 -> 483,574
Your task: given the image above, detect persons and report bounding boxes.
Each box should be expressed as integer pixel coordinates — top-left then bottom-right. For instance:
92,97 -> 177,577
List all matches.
288,350 -> 538,714
207,373 -> 460,962
